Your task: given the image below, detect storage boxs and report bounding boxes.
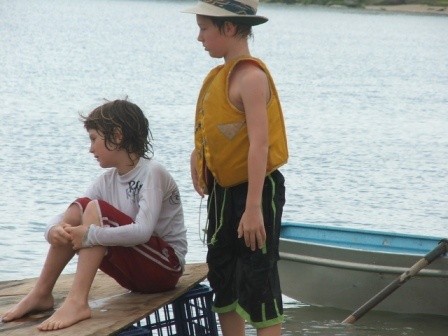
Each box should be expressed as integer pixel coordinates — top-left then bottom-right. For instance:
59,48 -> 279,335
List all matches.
114,284 -> 220,336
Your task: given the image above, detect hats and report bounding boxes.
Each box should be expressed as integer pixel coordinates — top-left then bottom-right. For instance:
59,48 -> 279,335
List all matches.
178,0 -> 268,25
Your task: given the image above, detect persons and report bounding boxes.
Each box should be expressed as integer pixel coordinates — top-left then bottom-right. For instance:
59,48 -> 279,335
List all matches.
180,0 -> 288,336
2,94 -> 188,331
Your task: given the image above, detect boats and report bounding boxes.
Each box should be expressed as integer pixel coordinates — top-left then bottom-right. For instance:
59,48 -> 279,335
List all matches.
277,223 -> 448,315
